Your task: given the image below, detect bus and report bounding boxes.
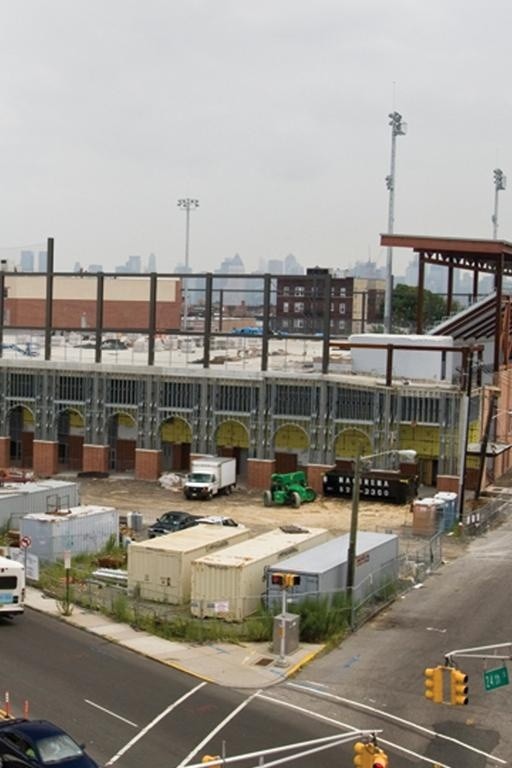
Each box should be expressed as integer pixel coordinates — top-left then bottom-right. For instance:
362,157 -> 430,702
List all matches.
0,555 -> 26,619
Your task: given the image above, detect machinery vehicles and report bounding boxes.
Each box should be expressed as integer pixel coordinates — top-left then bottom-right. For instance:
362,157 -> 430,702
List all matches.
264,471 -> 317,510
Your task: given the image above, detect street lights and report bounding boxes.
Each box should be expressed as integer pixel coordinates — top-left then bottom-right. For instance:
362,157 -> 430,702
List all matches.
380,110 -> 407,334
344,449 -> 418,625
491,168 -> 507,236
178,196 -> 200,330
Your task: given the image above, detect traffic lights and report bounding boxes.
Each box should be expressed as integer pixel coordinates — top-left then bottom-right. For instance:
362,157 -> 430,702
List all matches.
448,668 -> 469,708
423,666 -> 444,704
201,754 -> 220,768
269,572 -> 284,588
352,743 -> 371,768
370,752 -> 388,768
285,573 -> 301,588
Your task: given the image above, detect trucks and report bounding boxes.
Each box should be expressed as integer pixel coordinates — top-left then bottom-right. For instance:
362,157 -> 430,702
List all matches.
184,456 -> 237,501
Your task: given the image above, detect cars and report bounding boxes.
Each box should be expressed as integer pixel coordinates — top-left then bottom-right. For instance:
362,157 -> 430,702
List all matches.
147,510 -> 203,541
194,516 -> 245,531
0,715 -> 98,767
